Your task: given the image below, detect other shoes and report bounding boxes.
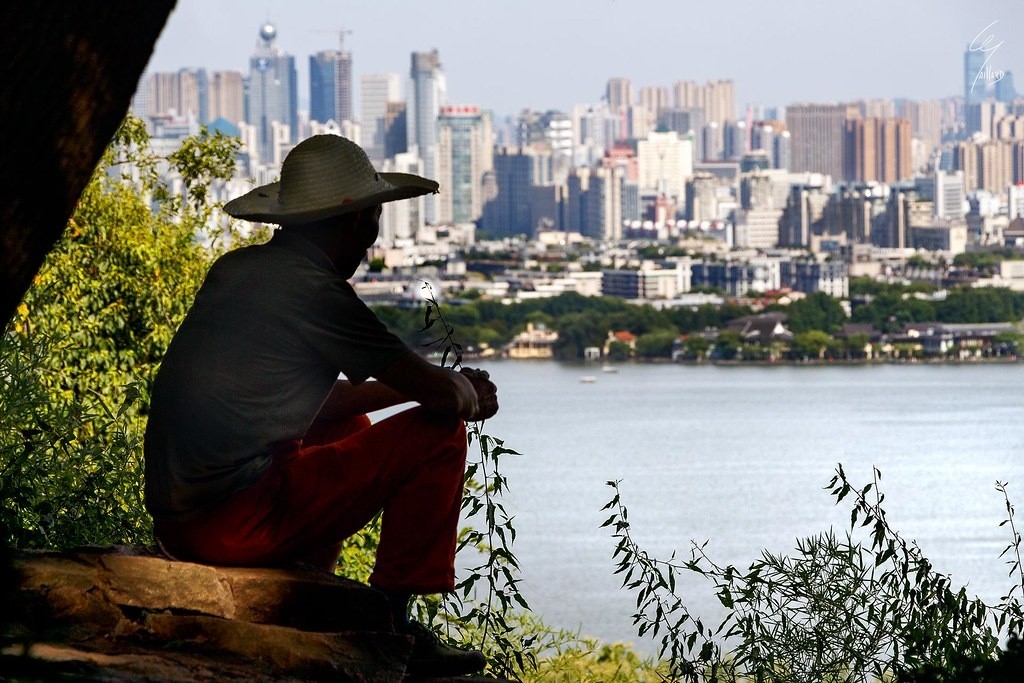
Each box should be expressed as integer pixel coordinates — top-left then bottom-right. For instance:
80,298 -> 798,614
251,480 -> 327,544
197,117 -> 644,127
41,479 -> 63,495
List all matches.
408,618 -> 487,679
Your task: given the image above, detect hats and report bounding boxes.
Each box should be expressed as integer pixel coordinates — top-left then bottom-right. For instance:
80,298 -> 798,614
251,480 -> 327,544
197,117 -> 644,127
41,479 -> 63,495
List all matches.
223,133 -> 441,224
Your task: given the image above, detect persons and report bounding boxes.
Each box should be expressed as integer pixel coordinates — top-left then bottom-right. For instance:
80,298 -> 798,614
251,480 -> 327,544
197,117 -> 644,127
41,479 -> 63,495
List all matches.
144,134 -> 499,676
